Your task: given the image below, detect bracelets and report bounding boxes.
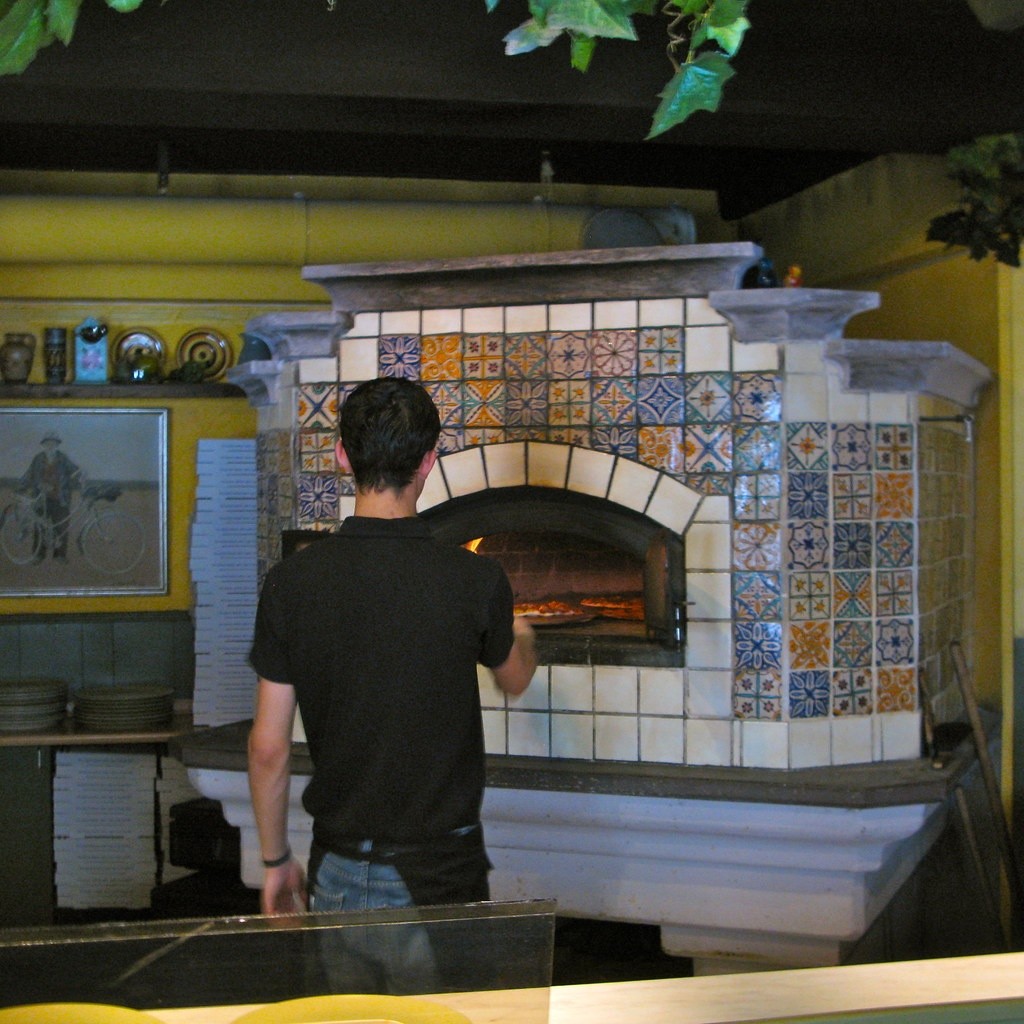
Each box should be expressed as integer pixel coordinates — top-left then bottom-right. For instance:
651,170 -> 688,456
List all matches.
261,848 -> 291,867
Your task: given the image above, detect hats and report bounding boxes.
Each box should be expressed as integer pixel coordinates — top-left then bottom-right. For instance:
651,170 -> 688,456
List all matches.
39,432 -> 61,449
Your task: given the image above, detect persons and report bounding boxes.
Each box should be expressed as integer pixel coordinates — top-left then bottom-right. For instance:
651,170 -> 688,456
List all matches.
248,375 -> 537,995
14,429 -> 82,564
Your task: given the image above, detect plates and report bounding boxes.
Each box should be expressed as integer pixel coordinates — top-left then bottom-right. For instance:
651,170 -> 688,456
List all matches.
514,610 -> 596,623
581,600 -> 636,610
234,995 -> 472,1024
0,1002 -> 168,1024
0,680 -> 67,731
75,684 -> 176,732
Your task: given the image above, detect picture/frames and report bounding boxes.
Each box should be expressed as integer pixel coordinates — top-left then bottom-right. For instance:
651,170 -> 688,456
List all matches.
0,408 -> 167,598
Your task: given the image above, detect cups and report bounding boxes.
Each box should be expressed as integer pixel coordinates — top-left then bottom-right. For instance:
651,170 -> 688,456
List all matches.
45,327 -> 66,385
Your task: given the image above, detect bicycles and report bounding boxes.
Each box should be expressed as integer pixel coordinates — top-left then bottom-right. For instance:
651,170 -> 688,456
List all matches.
0,479 -> 147,576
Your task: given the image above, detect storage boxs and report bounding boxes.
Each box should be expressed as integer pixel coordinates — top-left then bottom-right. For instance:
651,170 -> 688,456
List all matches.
53,747 -> 204,910
